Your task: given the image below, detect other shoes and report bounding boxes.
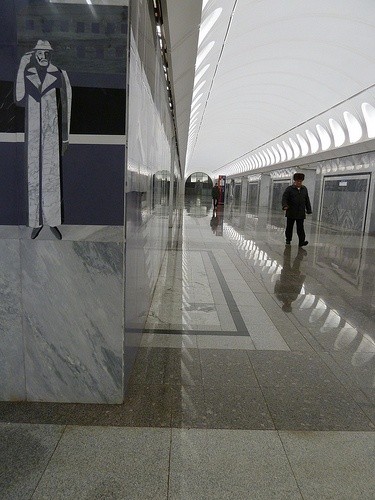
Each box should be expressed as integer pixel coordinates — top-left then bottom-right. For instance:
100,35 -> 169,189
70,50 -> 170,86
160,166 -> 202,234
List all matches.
286,241 -> 290,244
298,241 -> 308,246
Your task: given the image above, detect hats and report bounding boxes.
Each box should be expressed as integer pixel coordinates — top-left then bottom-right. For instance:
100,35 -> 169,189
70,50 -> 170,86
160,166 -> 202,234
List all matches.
294,173 -> 304,180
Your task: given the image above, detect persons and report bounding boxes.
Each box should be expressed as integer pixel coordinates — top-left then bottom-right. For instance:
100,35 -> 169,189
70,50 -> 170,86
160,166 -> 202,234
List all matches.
274,245 -> 307,312
212,183 -> 219,211
210,209 -> 219,233
282,173 -> 311,247
